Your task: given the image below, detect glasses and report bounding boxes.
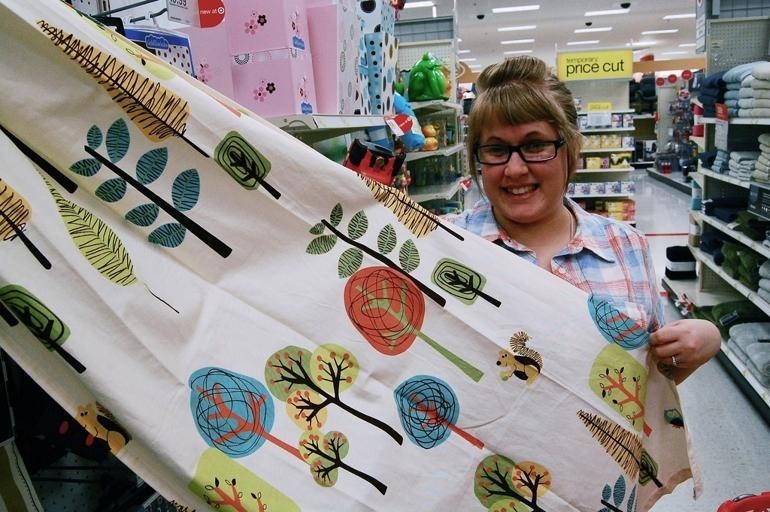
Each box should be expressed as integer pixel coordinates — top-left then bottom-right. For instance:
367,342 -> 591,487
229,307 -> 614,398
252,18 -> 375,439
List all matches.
472,139 -> 567,164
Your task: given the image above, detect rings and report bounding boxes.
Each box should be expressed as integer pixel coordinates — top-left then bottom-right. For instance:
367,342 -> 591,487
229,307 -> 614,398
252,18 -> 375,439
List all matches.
671,355 -> 678,367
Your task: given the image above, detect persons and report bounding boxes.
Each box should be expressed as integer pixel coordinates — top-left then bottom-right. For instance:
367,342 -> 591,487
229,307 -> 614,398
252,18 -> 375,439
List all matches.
433,53 -> 723,385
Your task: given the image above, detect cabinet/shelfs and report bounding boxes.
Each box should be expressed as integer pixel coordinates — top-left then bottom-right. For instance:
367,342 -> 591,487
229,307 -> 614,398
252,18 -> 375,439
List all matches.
404,98 -> 464,204
661,118 -> 770,427
566,108 -> 640,226
